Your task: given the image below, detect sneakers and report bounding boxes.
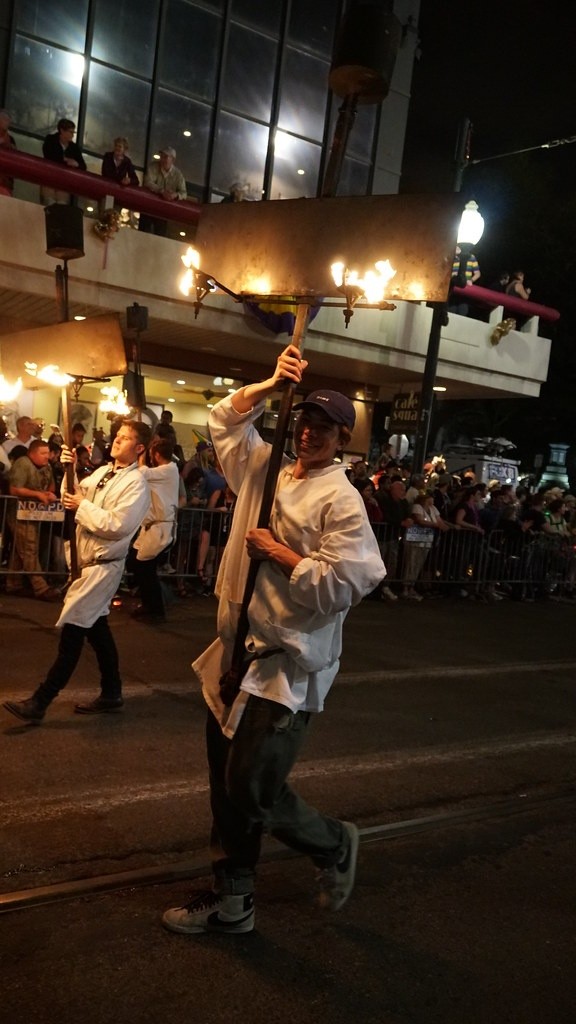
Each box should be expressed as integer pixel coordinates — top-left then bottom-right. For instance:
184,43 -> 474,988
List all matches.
162,890 -> 255,934
320,822 -> 359,912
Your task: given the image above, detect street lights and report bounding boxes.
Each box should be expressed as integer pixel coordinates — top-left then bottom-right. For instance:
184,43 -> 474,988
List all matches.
390,200 -> 484,579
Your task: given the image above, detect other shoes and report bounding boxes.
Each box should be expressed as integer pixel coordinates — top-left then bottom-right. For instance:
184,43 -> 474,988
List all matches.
381,587 -> 397,603
40,587 -> 61,600
403,591 -> 424,602
128,602 -> 165,622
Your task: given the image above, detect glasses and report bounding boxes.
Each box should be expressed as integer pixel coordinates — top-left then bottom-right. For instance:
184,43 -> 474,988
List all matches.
294,414 -> 342,433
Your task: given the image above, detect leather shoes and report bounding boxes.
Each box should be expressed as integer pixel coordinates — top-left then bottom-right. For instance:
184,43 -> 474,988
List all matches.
3,697 -> 45,725
76,694 -> 125,715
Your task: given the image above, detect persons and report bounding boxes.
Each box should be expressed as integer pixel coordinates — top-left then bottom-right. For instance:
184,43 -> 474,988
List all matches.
138,147 -> 186,235
494,272 -> 531,300
133,439 -> 181,623
1,419 -> 151,725
0,109 -> 18,197
163,344 -> 388,934
102,136 -> 138,214
345,444 -> 576,605
221,183 -> 244,203
154,410 -> 237,597
1,416 -> 108,600
449,247 -> 481,316
42,120 -> 86,205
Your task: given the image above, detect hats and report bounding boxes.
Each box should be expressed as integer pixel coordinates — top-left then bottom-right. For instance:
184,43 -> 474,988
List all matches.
292,392 -> 356,432
439,474 -> 452,485
565,494 -> 575,502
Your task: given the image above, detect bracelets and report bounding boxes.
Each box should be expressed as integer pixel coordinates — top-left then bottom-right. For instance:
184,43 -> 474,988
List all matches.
471,279 -> 473,282
